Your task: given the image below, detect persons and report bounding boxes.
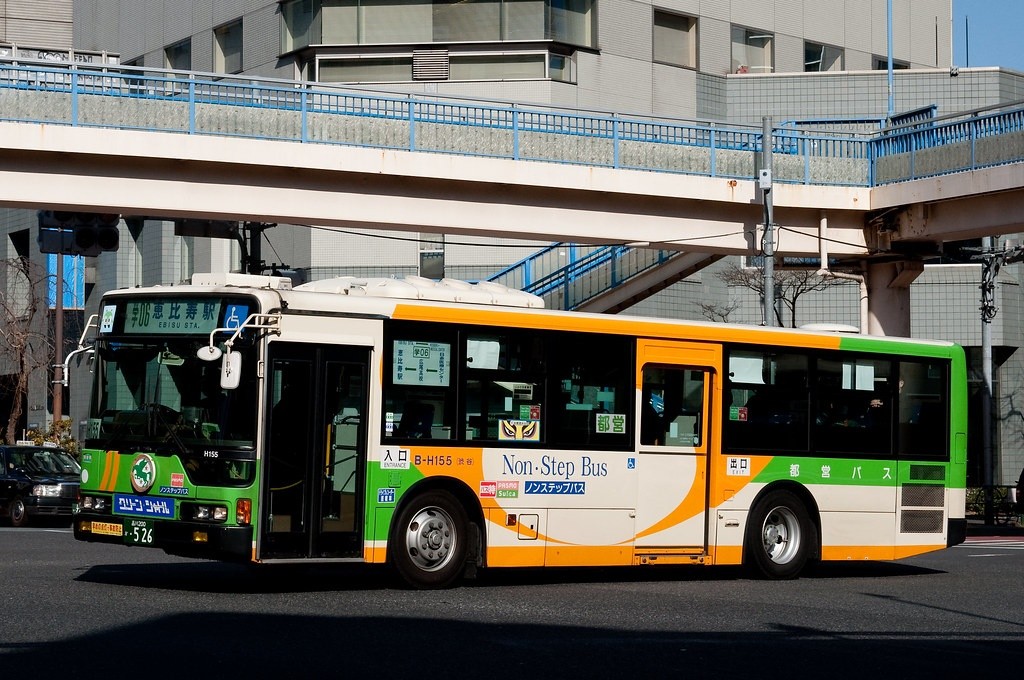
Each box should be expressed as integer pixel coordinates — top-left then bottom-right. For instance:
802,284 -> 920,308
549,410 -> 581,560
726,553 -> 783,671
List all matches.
7,457 -> 24,474
769,372 -> 913,434
195,374 -> 228,425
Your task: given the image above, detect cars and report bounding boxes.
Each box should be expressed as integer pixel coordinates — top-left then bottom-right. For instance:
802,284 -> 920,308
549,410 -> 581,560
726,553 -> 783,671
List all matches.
0,445 -> 84,527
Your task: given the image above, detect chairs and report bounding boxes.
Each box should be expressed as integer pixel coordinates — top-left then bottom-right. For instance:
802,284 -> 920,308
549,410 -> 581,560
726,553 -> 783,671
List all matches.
395,399 -> 435,439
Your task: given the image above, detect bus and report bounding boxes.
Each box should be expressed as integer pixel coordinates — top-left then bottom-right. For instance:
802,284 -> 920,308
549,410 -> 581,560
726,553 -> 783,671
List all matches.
50,270 -> 969,592
